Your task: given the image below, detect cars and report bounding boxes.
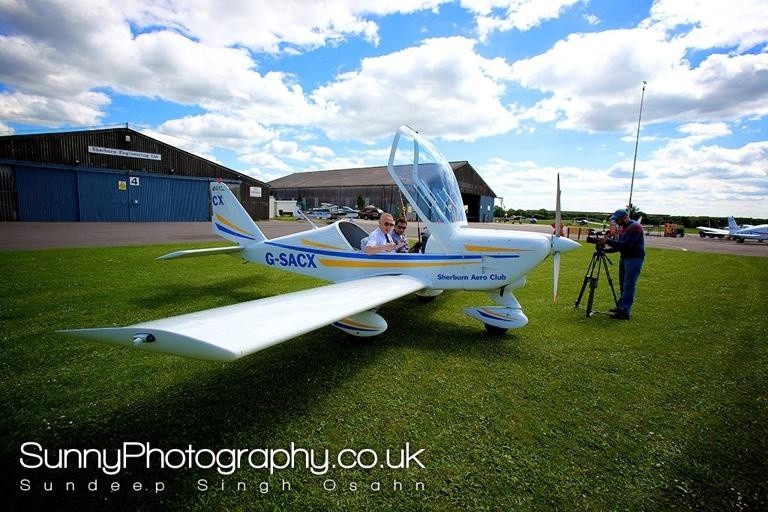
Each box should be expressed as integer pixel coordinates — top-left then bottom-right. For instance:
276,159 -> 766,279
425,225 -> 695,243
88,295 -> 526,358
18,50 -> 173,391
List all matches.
358,205 -> 382,220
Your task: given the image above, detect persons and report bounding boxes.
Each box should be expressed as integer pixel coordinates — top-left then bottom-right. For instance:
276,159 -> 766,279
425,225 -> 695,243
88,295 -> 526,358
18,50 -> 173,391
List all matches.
391,219 -> 410,253
595,209 -> 646,321
364,213 -> 396,255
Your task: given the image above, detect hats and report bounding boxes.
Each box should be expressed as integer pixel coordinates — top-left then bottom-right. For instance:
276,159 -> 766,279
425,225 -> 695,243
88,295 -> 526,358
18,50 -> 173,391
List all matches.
608,209 -> 629,221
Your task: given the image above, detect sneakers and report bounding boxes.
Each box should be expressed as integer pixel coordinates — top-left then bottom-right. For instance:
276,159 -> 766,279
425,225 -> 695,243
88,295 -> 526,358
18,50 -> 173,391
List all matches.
608,307 -> 631,320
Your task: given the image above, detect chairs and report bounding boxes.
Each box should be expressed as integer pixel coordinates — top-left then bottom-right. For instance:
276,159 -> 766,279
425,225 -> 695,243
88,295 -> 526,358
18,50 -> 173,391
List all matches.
360,236 -> 369,252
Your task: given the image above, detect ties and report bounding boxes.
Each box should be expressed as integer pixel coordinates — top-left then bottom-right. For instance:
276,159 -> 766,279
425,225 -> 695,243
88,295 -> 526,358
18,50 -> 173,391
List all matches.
384,233 -> 391,243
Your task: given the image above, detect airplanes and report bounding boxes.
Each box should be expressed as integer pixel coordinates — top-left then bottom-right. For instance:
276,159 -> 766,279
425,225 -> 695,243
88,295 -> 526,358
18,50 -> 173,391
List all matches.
55,124 -> 583,362
293,204 -> 358,221
704,218 -> 768,243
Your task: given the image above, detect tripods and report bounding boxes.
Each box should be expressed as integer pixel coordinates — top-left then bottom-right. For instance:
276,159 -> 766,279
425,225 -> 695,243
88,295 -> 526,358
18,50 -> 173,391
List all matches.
575,245 -> 618,317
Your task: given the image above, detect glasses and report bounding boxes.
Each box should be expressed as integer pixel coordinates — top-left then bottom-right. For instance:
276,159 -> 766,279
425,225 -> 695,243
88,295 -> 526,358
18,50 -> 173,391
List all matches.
382,221 -> 394,227
397,225 -> 407,230
